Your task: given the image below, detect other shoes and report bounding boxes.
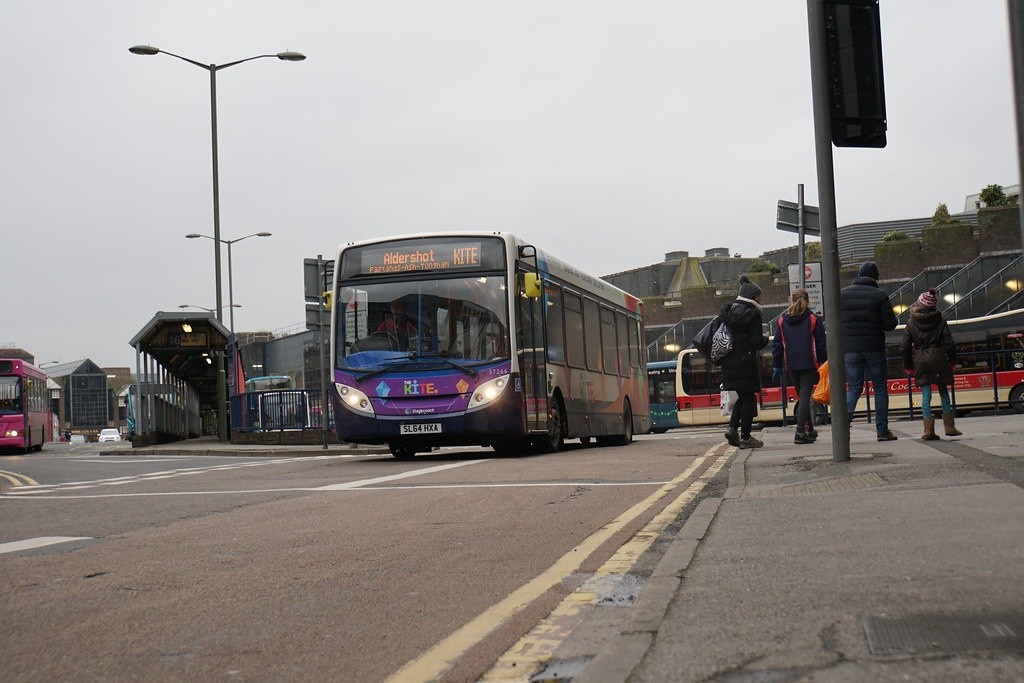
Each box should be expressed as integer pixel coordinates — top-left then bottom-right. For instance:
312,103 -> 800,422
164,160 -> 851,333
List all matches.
877,429 -> 898,441
740,438 -> 764,450
806,430 -> 818,439
725,427 -> 742,447
793,432 -> 814,444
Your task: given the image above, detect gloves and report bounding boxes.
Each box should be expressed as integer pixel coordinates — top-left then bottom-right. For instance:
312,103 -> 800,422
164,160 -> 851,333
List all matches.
906,368 -> 917,375
772,370 -> 784,383
949,362 -> 957,370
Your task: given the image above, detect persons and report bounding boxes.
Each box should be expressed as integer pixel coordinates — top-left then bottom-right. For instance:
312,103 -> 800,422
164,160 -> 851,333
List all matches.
839,262 -> 899,441
772,289 -> 828,444
903,289 -> 962,440
712,276 -> 770,449
375,295 -> 405,335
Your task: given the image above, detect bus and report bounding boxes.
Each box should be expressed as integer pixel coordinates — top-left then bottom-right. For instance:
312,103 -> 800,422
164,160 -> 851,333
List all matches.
322,233 -> 661,459
246,376 -> 311,433
0,358 -> 53,453
127,382 -> 178,434
646,362 -> 679,433
676,308 -> 1024,426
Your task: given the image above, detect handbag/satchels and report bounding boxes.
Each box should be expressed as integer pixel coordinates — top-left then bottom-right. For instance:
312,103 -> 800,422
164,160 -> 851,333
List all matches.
692,316 -> 733,366
813,361 -> 831,406
719,381 -> 739,415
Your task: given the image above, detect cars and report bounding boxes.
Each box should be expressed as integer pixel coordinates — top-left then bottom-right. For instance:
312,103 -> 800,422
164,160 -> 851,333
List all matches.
70,435 -> 87,443
97,428 -> 122,443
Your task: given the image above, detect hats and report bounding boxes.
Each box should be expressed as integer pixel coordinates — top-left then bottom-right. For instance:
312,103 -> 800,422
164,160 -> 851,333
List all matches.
739,275 -> 762,300
859,261 -> 880,281
916,287 -> 938,311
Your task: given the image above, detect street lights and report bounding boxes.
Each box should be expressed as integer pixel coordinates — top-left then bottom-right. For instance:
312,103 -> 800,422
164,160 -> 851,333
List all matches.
128,45 -> 307,441
185,232 -> 272,336
39,360 -> 58,369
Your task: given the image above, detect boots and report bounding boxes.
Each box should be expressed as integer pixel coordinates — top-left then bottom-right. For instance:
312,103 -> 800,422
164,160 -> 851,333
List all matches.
921,417 -> 940,440
942,413 -> 963,436
